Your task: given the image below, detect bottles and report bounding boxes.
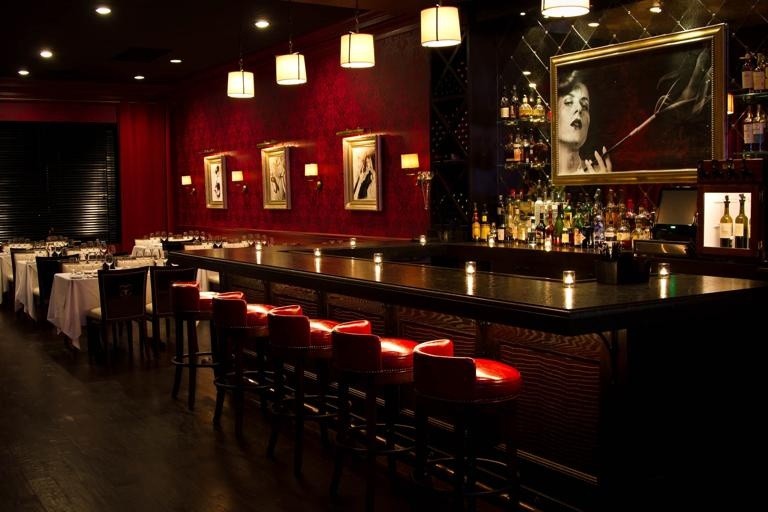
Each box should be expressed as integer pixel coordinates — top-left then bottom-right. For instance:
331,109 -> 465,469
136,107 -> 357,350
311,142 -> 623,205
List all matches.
471,84 -> 653,248
742,104 -> 768,158
741,53 -> 768,92
735,194 -> 748,248
720,196 -> 733,248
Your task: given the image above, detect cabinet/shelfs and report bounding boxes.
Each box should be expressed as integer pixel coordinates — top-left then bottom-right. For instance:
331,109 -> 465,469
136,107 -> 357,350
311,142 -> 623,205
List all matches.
498,117 -> 550,168
731,91 -> 768,156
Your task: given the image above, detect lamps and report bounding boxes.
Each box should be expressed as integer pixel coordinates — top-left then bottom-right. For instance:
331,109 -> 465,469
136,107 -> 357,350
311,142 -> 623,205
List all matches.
647,0 -> 664,14
401,153 -> 434,181
182,175 -> 195,192
540,1 -> 590,22
228,50 -> 256,99
274,38 -> 309,87
304,163 -> 322,190
417,2 -> 464,49
341,16 -> 375,71
232,171 -> 247,191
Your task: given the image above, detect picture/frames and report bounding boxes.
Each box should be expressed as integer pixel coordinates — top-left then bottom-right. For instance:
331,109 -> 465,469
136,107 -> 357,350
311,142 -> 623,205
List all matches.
204,154 -> 228,209
261,146 -> 292,210
342,133 -> 382,212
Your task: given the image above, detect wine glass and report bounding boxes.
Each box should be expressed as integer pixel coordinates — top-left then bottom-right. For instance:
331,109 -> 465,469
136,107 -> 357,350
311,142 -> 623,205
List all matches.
144,230 -> 268,248
7,237 -> 114,278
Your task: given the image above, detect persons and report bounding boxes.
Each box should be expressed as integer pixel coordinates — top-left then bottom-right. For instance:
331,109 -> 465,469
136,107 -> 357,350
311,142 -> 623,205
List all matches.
556,70 -> 613,175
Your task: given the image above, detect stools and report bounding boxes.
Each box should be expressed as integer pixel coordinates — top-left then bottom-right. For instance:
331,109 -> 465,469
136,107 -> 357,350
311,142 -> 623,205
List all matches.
267,303 -> 338,477
174,286 -> 248,408
212,295 -> 277,442
411,334 -> 519,512
332,319 -> 415,507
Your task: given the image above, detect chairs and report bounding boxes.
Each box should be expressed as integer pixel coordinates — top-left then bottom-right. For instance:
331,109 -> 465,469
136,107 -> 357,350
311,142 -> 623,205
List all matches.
0,238 -> 198,368
